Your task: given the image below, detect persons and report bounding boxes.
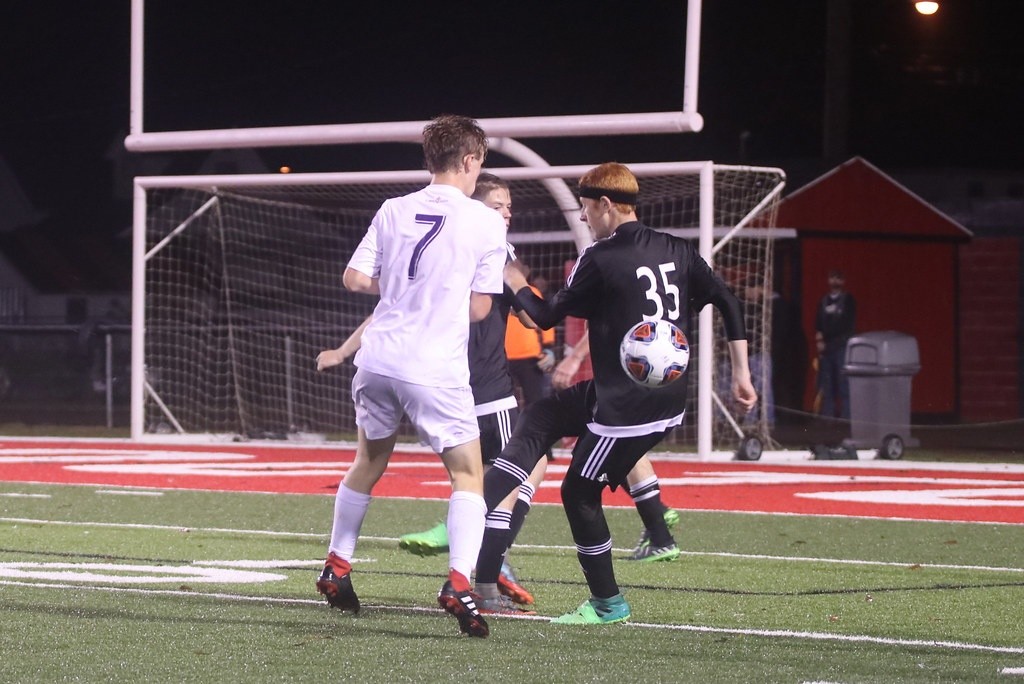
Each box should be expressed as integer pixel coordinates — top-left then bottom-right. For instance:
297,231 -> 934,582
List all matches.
503,164 -> 758,626
315,114 -> 857,635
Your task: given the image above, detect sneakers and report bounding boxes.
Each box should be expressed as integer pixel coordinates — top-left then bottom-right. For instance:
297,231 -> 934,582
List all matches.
497,562 -> 533,605
617,535 -> 681,563
549,594 -> 632,624
398,522 -> 449,556
436,581 -> 489,639
639,507 -> 679,546
316,565 -> 361,613
471,590 -> 538,616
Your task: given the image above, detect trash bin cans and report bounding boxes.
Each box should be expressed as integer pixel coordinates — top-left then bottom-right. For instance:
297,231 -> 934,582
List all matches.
839,330 -> 922,461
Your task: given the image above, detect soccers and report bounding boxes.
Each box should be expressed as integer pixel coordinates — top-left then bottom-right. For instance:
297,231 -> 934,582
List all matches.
619,318 -> 690,388
